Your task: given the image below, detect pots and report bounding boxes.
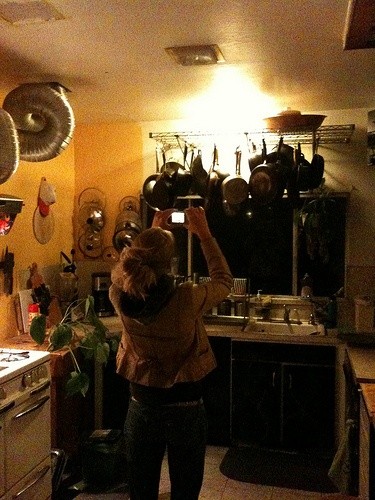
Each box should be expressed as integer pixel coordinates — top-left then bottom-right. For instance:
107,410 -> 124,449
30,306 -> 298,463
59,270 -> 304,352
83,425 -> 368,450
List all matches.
143,138 -> 325,223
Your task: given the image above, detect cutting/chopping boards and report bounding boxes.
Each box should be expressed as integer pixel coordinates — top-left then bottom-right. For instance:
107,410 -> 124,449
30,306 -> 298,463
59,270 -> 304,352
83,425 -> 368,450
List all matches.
15,289 -> 33,335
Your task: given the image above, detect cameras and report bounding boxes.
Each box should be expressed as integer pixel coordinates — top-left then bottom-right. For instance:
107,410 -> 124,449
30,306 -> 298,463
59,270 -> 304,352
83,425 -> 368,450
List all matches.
172,212 -> 189,225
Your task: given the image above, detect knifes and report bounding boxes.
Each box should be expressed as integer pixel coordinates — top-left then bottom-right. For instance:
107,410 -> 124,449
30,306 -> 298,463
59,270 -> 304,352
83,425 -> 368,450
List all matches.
32,283 -> 51,316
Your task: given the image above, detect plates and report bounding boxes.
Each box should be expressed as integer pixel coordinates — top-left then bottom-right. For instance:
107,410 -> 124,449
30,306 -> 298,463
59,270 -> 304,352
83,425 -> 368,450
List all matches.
232,294 -> 252,297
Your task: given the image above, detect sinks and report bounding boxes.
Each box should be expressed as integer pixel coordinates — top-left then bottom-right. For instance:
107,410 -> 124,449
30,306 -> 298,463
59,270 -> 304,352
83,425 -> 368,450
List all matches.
243,322 -> 326,337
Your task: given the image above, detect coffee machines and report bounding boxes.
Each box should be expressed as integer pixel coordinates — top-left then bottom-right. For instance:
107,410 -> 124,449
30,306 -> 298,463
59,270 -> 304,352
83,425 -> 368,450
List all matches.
92,272 -> 114,318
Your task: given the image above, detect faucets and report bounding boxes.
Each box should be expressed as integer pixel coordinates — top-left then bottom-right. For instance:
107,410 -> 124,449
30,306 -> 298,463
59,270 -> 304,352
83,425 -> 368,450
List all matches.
283,304 -> 290,322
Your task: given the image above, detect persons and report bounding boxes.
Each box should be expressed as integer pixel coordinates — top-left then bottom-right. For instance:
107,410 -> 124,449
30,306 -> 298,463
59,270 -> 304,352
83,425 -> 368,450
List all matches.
109,206 -> 234,500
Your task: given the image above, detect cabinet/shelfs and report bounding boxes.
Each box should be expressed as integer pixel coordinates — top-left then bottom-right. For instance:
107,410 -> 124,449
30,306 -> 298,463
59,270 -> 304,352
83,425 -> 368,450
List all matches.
229,340 -> 339,456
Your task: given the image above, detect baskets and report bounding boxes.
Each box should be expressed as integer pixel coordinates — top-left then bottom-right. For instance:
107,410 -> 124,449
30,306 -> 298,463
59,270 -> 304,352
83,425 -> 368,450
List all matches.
198,276 -> 247,294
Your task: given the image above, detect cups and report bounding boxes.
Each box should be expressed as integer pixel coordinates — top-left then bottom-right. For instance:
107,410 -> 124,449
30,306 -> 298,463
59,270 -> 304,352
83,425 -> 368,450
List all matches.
217,299 -> 231,316
234,278 -> 247,294
235,301 -> 248,316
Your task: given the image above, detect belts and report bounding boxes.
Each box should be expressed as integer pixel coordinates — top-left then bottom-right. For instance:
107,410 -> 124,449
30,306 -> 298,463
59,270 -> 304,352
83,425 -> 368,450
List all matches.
131,395 -> 203,407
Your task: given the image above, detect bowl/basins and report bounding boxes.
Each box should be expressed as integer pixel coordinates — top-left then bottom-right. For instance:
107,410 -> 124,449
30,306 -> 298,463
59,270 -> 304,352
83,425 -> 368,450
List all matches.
263,115 -> 328,133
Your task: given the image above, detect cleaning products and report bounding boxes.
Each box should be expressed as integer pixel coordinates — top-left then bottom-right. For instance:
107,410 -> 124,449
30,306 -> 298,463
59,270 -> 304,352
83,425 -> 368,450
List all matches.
254,289 -> 263,317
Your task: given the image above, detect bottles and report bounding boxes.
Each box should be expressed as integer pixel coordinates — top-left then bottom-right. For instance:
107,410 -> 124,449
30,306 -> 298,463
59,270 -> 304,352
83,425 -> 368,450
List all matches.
324,296 -> 337,329
28,302 -> 39,336
